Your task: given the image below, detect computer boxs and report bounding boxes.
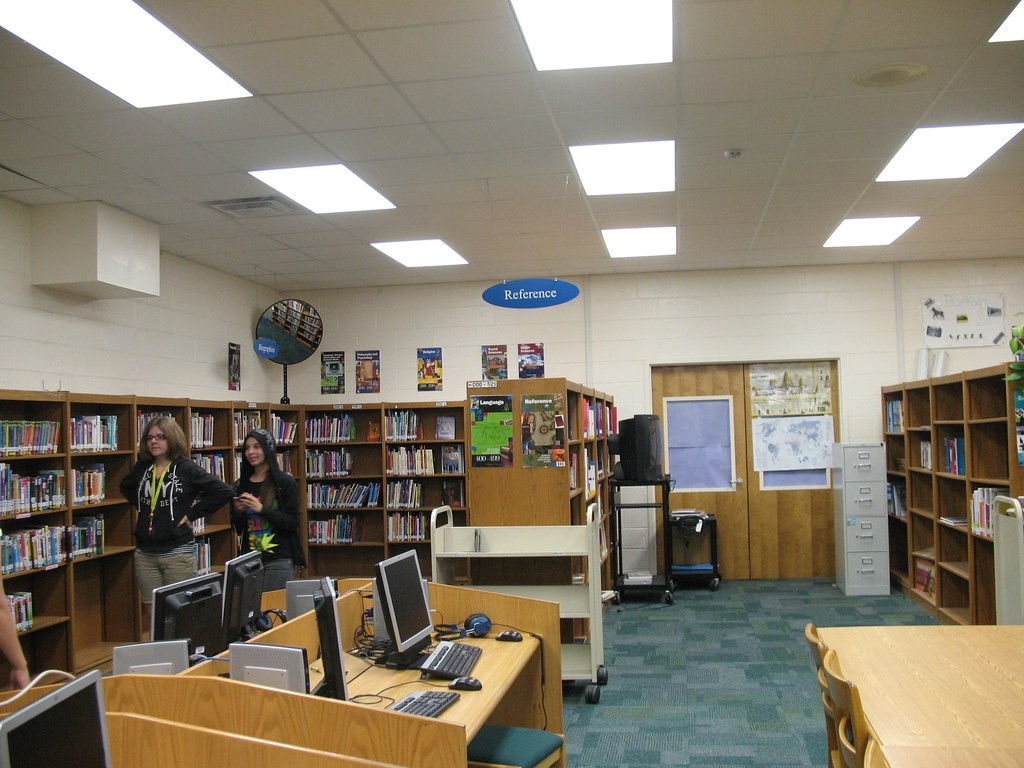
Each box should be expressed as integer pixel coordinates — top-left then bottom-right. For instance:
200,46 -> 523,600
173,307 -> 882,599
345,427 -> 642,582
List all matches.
228,642 -> 310,694
112,638 -> 192,676
374,578 -> 429,642
286,579 -> 340,622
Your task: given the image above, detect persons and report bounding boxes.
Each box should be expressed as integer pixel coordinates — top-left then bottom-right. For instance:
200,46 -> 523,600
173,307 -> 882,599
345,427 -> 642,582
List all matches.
119,416 -> 233,617
229,429 -> 307,593
0,553 -> 30,692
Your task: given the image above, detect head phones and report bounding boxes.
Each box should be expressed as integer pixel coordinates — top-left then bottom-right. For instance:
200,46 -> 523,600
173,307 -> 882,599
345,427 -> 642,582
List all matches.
434,613 -> 492,641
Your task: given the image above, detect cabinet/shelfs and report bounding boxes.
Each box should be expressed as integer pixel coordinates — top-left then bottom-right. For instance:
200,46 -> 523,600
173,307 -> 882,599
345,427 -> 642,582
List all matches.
882,363 -> 1022,627
832,441 -> 890,597
469,379 -> 614,640
0,387 -> 471,675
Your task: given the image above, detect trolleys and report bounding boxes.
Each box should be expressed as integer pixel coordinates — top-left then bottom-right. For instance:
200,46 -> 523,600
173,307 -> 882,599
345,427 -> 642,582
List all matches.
605,473 -> 675,606
670,512 -> 722,593
428,501 -> 609,706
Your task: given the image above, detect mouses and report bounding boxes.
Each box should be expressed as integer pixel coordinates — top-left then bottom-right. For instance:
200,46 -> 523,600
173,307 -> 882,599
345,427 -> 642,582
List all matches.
448,677 -> 482,692
495,630 -> 523,642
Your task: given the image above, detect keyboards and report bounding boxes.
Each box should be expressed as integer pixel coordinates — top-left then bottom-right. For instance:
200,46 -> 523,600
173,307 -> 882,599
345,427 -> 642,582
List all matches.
421,641 -> 482,678
389,691 -> 461,718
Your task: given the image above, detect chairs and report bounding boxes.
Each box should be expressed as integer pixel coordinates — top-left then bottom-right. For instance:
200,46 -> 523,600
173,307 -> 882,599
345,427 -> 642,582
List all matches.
803,621 -> 879,768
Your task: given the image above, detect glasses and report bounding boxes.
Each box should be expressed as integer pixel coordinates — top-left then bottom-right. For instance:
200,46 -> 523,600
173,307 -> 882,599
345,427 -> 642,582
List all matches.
146,434 -> 167,440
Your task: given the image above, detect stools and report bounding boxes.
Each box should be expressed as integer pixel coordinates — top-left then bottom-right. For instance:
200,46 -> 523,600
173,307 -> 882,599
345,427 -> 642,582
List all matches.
467,721 -> 565,768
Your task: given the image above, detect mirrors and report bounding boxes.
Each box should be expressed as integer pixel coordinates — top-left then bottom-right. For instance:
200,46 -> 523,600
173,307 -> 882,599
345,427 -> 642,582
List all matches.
256,299 -> 323,365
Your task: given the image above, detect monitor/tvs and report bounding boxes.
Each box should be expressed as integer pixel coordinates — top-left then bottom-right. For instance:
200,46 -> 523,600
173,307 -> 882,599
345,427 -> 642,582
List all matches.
308,577 -> 348,702
609,415 -> 663,480
151,572 -> 229,667
0,670 -> 114,768
373,549 -> 433,669
221,549 -> 273,642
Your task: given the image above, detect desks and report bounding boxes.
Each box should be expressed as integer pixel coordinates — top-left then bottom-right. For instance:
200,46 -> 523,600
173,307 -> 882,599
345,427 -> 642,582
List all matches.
0,578 -> 564,767
822,627 -> 1024,768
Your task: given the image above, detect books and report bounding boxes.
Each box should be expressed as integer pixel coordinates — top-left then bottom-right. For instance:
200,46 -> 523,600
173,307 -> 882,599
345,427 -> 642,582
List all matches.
384,409 -> 434,542
0,416 -> 118,575
940,516 -> 967,526
234,410 -> 297,482
599,522 -> 608,562
944,436 -> 966,476
6,592 -> 33,633
887,482 -> 907,517
441,445 -> 464,474
916,558 -> 935,600
441,477 -> 465,508
601,591 -> 615,601
920,441 -> 932,471
304,414 -> 381,544
970,488 -> 1010,538
139,411 -> 225,575
568,399 -> 617,499
886,400 -> 903,432
436,416 -> 455,440
